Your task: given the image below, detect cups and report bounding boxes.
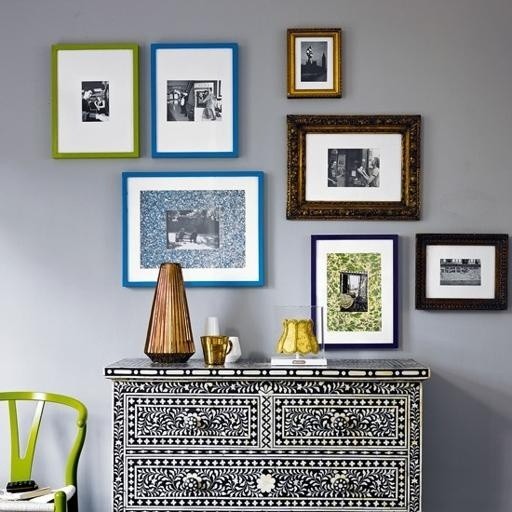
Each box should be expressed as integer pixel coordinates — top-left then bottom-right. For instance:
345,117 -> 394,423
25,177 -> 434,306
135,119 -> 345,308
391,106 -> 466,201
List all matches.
200,335 -> 232,365
205,317 -> 220,336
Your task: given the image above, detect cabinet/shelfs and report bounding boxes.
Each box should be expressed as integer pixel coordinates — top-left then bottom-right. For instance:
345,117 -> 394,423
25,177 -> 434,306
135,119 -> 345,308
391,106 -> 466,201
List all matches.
103,357 -> 431,512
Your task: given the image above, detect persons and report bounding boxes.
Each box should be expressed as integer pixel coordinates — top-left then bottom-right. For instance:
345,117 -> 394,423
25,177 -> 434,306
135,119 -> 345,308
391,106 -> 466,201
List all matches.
339,282 -> 368,312
94,96 -> 107,116
82,88 -> 94,113
176,228 -> 197,243
328,160 -> 343,187
357,157 -> 379,187
201,90 -> 216,121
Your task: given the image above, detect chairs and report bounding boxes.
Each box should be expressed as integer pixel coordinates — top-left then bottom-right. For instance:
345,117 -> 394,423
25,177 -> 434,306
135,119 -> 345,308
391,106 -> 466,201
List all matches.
0,390 -> 88,512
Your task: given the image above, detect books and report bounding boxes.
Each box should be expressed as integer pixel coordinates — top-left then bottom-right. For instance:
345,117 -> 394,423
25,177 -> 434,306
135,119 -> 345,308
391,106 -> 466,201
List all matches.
0,487 -> 51,501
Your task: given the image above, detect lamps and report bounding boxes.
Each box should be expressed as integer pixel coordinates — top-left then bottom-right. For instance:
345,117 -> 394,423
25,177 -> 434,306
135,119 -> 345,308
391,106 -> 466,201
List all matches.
414,231 -> 508,311
149,41 -> 239,159
120,170 -> 266,287
286,113 -> 422,222
286,27 -> 343,99
309,233 -> 400,350
48,41 -> 140,159
270,306 -> 327,369
